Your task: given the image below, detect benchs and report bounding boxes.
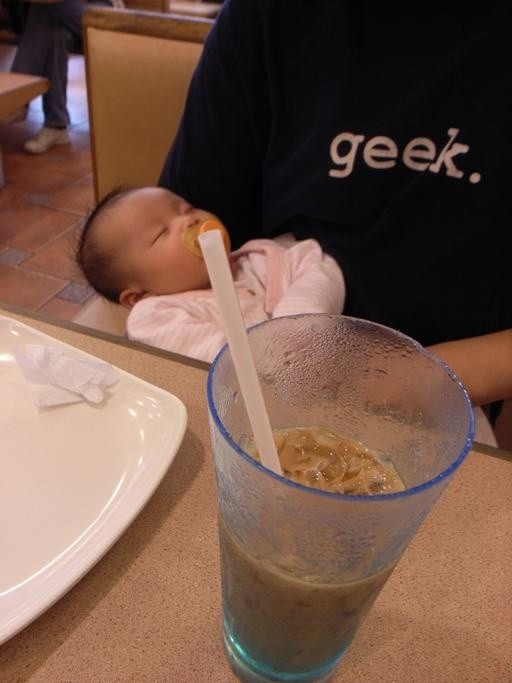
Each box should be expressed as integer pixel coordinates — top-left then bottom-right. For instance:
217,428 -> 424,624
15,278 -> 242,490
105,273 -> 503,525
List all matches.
69,5 -> 213,337
0,70 -> 54,189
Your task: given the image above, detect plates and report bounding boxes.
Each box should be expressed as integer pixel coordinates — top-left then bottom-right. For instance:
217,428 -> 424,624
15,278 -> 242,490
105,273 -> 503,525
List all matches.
0,315 -> 188,649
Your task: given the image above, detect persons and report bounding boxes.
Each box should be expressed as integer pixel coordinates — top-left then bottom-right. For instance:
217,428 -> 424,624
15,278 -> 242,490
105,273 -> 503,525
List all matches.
1,0 -> 116,155
146,0 -> 512,429
73,179 -> 354,378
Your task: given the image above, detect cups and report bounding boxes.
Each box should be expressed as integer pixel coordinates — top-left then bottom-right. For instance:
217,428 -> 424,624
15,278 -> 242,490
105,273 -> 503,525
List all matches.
204,310 -> 478,683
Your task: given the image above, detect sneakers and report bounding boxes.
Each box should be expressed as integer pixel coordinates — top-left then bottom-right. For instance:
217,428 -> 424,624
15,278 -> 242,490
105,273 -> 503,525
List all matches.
24,127 -> 69,153
